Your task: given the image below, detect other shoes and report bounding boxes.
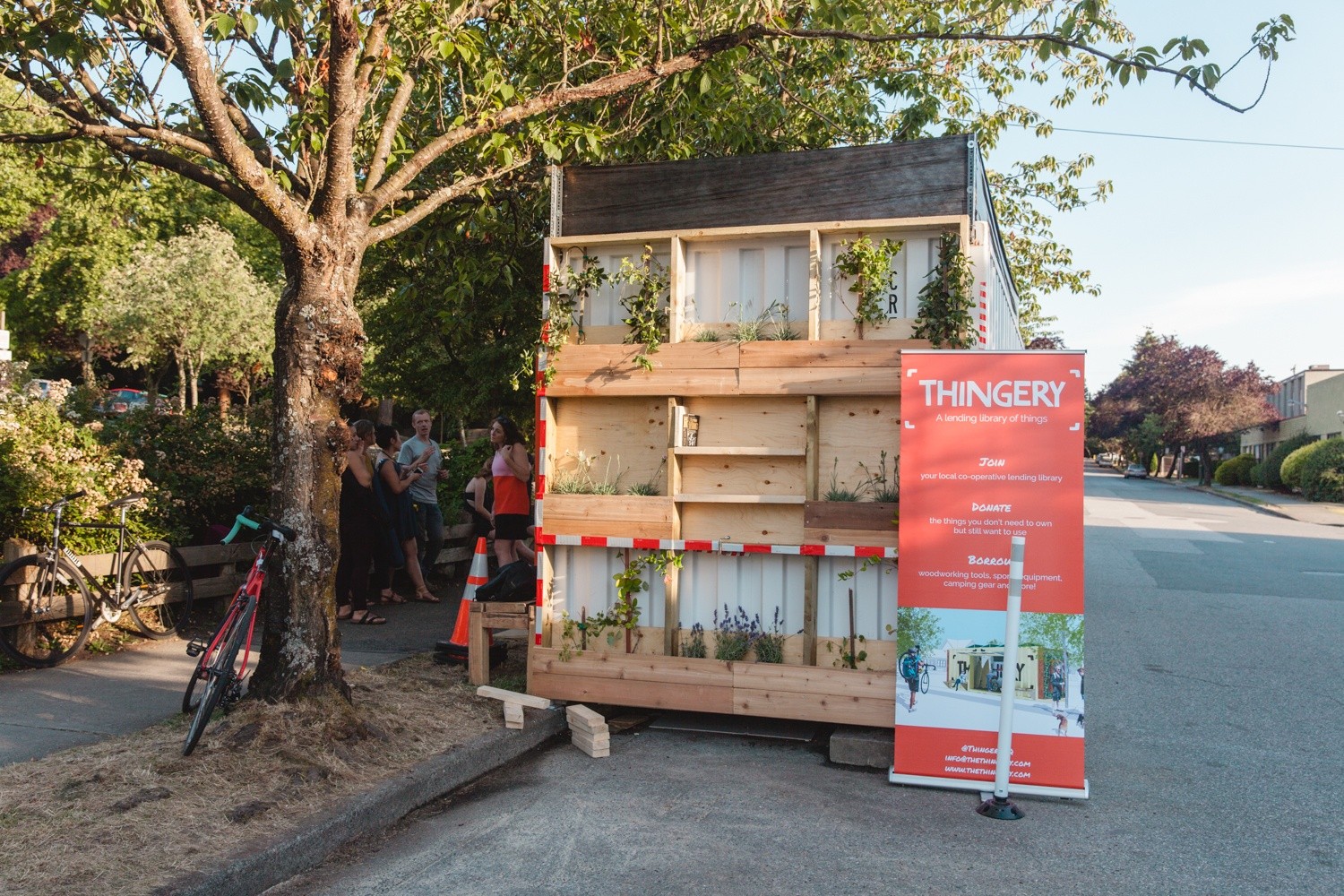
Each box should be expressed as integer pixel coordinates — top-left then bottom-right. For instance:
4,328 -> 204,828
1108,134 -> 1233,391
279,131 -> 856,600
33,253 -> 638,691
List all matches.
956,688 -> 958,691
908,706 -> 914,713
952,685 -> 955,687
913,700 -> 918,705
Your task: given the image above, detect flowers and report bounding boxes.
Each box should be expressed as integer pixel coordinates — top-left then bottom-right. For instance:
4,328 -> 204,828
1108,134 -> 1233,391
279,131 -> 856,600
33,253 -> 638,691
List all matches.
676,602 -> 805,663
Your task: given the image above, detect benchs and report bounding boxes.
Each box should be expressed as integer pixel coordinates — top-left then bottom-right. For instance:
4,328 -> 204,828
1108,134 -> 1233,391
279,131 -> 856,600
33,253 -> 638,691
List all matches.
469,599 -> 535,685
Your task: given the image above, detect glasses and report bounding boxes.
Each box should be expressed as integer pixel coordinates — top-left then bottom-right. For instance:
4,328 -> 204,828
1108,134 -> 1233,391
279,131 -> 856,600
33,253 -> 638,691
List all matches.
1058,669 -> 1061,670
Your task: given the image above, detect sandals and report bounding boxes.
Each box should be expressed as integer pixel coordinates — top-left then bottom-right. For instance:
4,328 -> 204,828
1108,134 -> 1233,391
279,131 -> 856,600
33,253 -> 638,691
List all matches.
415,586 -> 441,603
351,610 -> 386,624
366,601 -> 376,606
380,591 -> 408,603
336,604 -> 353,619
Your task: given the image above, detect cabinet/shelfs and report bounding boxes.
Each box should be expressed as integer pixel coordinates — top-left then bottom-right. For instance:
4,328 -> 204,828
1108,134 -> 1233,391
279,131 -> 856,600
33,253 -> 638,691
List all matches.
676,446 -> 809,503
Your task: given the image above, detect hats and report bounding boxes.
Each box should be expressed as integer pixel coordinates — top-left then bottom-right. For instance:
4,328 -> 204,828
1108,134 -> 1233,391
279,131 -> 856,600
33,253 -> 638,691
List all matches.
908,648 -> 916,653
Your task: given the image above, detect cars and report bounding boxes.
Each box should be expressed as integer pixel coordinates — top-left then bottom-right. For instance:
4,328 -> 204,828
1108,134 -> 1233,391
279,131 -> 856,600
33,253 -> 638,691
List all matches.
1096,453 -> 1109,463
1123,463 -> 1148,480
22,378 -> 65,402
94,388 -> 169,423
1099,456 -> 1113,468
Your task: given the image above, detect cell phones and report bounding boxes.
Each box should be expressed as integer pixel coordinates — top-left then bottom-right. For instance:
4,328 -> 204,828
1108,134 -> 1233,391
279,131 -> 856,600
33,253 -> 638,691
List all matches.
414,467 -> 423,473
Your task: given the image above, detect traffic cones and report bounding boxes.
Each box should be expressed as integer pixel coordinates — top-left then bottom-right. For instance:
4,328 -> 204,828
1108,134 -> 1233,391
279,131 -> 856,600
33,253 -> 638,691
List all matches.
432,536 -> 502,674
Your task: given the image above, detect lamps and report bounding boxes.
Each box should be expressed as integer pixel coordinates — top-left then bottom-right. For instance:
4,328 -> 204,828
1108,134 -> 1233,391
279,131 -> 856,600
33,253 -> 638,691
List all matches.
1287,400 -> 1308,408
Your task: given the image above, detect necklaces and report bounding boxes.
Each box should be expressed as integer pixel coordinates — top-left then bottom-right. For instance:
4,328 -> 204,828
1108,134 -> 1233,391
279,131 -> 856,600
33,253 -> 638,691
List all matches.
381,450 -> 392,460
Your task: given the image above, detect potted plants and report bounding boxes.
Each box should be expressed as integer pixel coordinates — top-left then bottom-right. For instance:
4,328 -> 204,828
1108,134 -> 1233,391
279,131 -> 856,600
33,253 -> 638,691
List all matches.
508,232 -> 982,394
541,448 -> 673,524
533,549 -> 898,699
805,450 -> 899,531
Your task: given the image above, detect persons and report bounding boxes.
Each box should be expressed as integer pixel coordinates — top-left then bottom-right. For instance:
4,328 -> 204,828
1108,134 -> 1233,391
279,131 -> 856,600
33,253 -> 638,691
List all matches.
375,424 -> 441,603
347,419 -> 408,606
337,422 -> 386,625
1077,668 -> 1084,699
490,416 -> 529,568
463,457 -> 534,563
902,647 -> 925,712
952,669 -> 966,691
397,409 -> 449,592
1051,665 -> 1065,711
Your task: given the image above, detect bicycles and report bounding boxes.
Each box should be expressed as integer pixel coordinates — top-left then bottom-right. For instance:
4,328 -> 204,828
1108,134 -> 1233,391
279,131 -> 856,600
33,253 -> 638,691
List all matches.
181,504 -> 298,757
0,489 -> 194,668
